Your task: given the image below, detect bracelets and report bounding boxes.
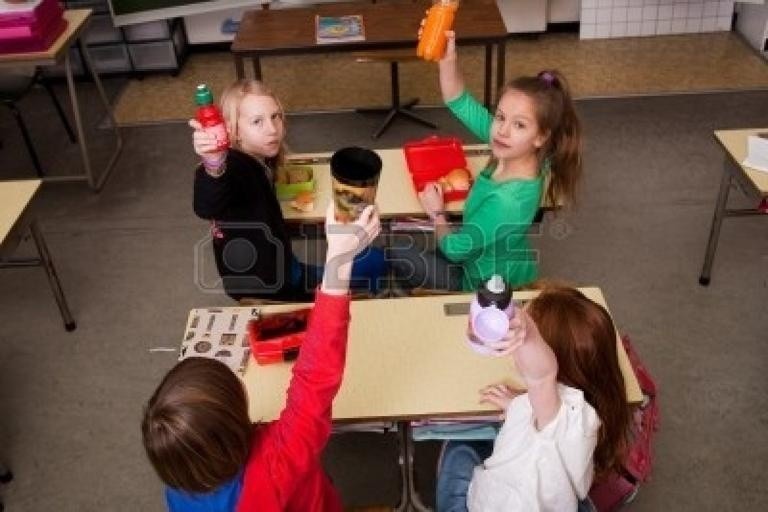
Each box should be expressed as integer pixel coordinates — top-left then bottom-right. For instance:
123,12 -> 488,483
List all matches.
204,160 -> 226,174
433,211 -> 448,217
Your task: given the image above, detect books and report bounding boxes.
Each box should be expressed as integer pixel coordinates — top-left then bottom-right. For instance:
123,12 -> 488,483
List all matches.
315,14 -> 366,44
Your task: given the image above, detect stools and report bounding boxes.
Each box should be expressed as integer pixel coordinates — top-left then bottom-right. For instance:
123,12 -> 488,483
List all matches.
323,432 -> 410,512
351,43 -> 439,140
404,435 -> 495,512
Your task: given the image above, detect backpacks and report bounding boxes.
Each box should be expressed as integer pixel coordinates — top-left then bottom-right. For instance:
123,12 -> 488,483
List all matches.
585,332 -> 660,511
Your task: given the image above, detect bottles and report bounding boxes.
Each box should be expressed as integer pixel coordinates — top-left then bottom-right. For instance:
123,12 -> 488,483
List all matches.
464,276 -> 516,357
193,82 -> 230,155
415,0 -> 461,64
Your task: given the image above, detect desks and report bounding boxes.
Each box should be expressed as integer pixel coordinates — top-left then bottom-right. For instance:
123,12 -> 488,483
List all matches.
226,1 -> 510,120
695,125 -> 766,289
0,7 -> 133,198
0,177 -> 78,483
173,285 -> 646,428
258,145 -> 566,239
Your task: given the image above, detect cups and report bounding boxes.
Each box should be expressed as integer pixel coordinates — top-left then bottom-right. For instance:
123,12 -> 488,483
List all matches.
329,146 -> 381,223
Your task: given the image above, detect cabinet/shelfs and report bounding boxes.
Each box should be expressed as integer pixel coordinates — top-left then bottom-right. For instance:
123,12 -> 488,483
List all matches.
0,0 -> 195,80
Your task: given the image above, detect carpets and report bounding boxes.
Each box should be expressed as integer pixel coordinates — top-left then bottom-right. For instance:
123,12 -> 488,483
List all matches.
95,26 -> 766,130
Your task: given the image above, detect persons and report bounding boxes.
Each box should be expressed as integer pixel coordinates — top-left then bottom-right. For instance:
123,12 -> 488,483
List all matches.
142,199 -> 384,511
386,10 -> 583,291
433,286 -> 630,512
188,77 -> 390,301
